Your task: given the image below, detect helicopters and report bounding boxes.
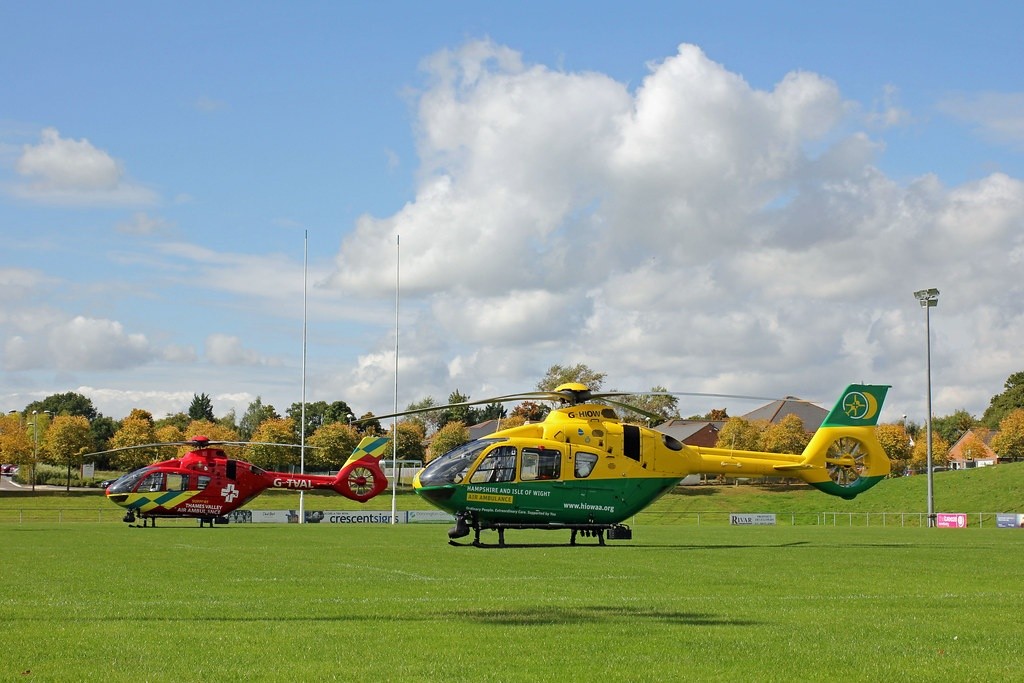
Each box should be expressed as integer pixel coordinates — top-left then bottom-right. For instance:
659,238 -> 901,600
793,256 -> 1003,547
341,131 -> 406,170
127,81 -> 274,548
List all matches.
82,435 -> 393,529
350,382 -> 892,547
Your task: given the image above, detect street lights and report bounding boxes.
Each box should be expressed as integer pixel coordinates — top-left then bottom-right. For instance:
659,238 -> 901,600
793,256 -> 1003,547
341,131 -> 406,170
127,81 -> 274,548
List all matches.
32,410 -> 38,492
903,415 -> 908,437
914,285 -> 941,527
9,409 -> 21,431
44,410 -> 55,426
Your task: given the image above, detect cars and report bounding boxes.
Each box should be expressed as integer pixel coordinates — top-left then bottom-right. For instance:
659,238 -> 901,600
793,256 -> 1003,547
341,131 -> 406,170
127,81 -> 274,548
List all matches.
0,463 -> 20,474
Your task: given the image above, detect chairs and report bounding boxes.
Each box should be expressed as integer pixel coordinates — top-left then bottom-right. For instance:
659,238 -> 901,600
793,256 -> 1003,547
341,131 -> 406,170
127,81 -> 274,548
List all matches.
485,462 -> 497,482
504,456 -> 516,480
